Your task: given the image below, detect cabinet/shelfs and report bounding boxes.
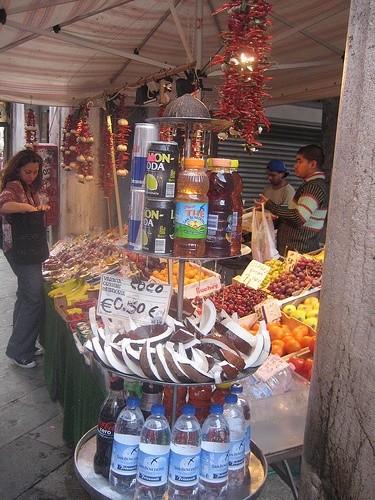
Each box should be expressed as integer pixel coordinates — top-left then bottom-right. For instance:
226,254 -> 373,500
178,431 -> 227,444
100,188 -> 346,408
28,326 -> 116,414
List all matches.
74,116 -> 272,500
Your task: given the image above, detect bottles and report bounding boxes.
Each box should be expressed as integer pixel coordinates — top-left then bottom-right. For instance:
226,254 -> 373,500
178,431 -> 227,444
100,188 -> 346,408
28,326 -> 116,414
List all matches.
143,381 -> 188,421
231,382 -> 251,467
223,395 -> 245,489
108,396 -> 145,493
93,376 -> 128,477
188,382 -> 230,419
231,160 -> 244,256
115,374 -> 143,399
197,404 -> 229,497
205,159 -> 233,258
168,404 -> 202,499
136,403 -> 172,499
206,158 -> 212,175
173,158 -> 209,258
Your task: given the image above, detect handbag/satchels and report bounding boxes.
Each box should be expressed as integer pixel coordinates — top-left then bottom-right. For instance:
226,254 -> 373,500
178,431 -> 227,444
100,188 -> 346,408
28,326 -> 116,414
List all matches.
250,202 -> 278,264
4,179 -> 49,266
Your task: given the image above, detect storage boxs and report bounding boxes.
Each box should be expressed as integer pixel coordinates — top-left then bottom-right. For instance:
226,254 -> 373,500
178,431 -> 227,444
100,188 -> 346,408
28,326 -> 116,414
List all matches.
150,262 -> 322,362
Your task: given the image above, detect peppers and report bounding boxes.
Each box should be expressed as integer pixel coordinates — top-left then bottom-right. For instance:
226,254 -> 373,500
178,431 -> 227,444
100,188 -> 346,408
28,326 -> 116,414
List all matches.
161,0 -> 276,158
24,108 -> 40,154
61,116 -> 130,197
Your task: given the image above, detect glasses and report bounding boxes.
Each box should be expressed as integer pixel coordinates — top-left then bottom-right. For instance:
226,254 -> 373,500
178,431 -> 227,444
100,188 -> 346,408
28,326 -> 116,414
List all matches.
265,172 -> 277,177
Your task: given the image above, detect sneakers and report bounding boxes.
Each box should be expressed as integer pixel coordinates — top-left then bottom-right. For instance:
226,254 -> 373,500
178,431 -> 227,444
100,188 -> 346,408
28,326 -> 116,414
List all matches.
34,347 -> 42,356
6,351 -> 36,369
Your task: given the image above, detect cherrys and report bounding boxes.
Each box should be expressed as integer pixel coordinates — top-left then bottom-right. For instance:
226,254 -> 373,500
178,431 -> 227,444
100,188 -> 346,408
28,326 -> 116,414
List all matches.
193,282 -> 266,320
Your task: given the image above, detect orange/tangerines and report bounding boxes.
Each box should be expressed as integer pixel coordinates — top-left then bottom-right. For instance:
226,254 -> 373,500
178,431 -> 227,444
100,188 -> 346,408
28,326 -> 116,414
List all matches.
244,322 -> 310,356
150,261 -> 207,289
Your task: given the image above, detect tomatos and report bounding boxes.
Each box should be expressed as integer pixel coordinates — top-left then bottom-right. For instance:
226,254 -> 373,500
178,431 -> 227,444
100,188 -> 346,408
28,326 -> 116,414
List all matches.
288,338 -> 316,381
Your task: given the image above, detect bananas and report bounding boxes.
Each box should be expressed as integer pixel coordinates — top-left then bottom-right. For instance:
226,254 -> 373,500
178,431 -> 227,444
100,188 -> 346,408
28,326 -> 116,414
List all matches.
48,274 -> 90,305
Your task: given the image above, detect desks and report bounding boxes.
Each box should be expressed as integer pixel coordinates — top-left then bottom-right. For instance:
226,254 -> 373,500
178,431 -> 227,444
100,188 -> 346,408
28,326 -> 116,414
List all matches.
39,281 -> 310,500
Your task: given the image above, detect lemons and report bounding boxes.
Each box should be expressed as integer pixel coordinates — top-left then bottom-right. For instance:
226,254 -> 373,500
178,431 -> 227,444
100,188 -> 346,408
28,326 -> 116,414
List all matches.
282,296 -> 319,332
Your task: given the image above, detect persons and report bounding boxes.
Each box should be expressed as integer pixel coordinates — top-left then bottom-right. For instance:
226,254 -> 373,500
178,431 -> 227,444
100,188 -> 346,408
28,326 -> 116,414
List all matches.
0,149 -> 50,368
253,145 -> 330,258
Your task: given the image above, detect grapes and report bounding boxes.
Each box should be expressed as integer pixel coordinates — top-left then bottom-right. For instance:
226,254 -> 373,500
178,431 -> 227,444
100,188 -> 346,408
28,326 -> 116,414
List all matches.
258,256 -> 324,299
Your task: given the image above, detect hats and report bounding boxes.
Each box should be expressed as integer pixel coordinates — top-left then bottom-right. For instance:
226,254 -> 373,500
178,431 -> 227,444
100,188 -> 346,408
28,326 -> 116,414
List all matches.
267,159 -> 288,172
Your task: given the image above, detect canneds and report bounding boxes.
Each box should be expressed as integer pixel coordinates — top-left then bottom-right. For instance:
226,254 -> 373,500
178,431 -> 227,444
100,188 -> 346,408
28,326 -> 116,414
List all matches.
126,122 -> 179,255
